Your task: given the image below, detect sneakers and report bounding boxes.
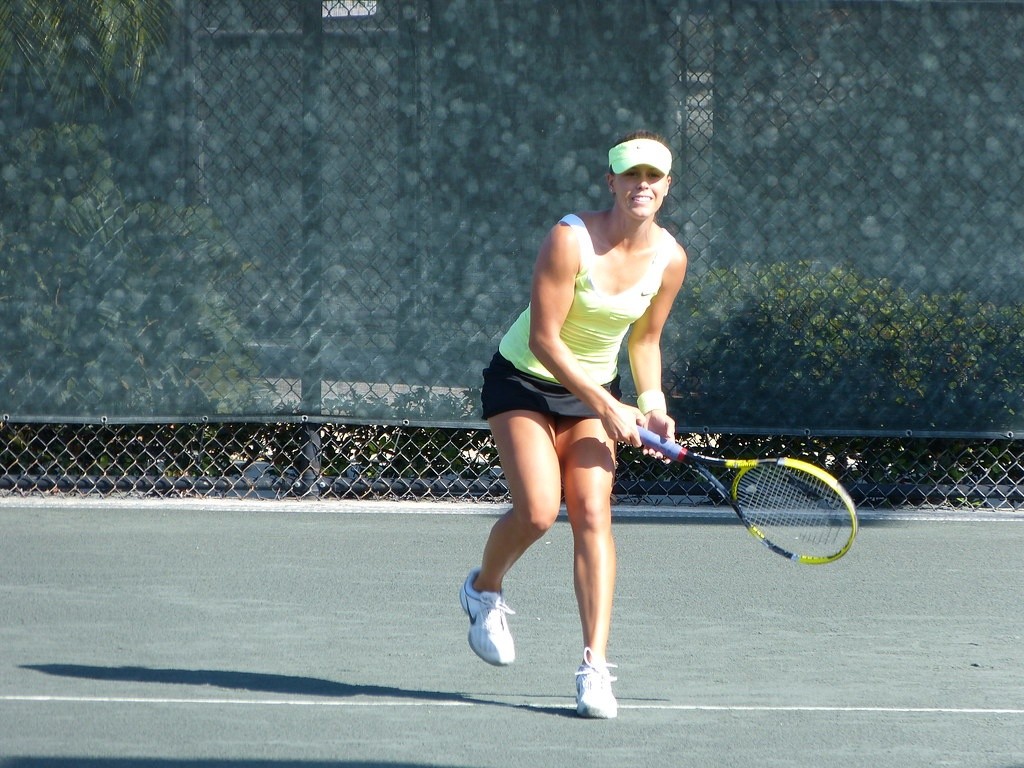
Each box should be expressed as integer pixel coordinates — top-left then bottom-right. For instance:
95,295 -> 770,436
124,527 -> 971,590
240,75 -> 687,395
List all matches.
460,565 -> 519,666
575,645 -> 620,719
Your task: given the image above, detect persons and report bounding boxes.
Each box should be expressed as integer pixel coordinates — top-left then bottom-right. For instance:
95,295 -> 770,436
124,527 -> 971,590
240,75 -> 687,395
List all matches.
461,130 -> 687,719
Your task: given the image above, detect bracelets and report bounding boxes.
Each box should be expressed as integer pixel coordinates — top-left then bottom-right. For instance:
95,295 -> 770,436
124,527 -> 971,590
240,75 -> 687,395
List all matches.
637,390 -> 667,416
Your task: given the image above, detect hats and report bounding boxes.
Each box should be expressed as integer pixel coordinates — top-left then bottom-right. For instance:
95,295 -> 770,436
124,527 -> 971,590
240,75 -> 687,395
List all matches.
609,139 -> 673,180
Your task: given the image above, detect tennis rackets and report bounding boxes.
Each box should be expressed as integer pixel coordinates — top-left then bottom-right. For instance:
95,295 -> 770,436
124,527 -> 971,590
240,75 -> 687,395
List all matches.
636,423 -> 859,565
